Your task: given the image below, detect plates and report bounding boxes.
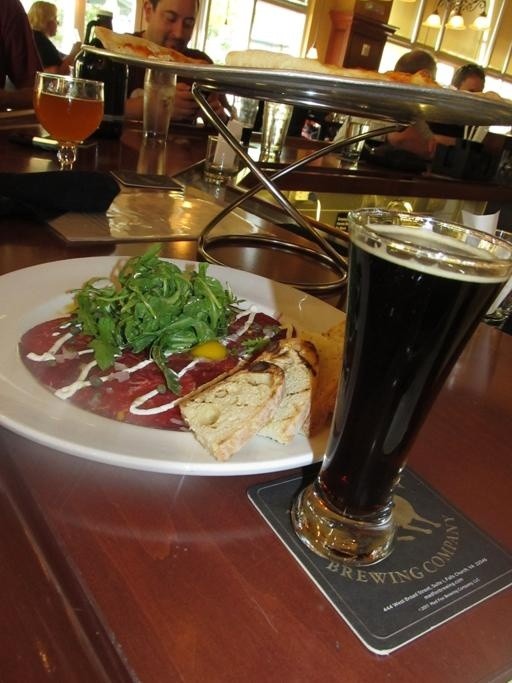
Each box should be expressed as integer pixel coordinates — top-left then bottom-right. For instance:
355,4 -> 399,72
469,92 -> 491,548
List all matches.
0,254 -> 344,478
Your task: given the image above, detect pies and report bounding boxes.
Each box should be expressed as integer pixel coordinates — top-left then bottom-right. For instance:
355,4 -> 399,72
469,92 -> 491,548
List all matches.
92,26 -> 209,64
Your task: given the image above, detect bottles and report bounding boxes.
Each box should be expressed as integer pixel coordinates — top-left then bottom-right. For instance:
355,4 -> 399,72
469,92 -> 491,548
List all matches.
75,11 -> 126,111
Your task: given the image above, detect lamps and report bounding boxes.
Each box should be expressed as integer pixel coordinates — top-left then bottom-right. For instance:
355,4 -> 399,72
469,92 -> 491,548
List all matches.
421,1 -> 492,33
306,2 -> 324,60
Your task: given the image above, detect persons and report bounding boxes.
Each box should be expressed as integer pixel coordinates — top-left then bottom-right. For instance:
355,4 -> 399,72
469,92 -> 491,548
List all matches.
124,0 -> 229,129
426,63 -> 485,145
332,50 -> 437,158
28,1 -> 81,96
0,0 -> 45,112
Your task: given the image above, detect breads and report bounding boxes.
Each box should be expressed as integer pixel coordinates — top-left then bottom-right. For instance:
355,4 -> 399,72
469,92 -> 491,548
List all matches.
178,321 -> 346,462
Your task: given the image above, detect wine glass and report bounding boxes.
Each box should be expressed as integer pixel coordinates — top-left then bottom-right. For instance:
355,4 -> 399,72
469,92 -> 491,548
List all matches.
366,121 -> 389,157
29,71 -> 108,171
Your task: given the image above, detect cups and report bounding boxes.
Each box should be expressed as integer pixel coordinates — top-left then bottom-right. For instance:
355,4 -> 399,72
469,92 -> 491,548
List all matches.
229,95 -> 291,160
142,68 -> 174,144
292,205 -> 512,567
200,137 -> 239,182
337,113 -> 368,162
